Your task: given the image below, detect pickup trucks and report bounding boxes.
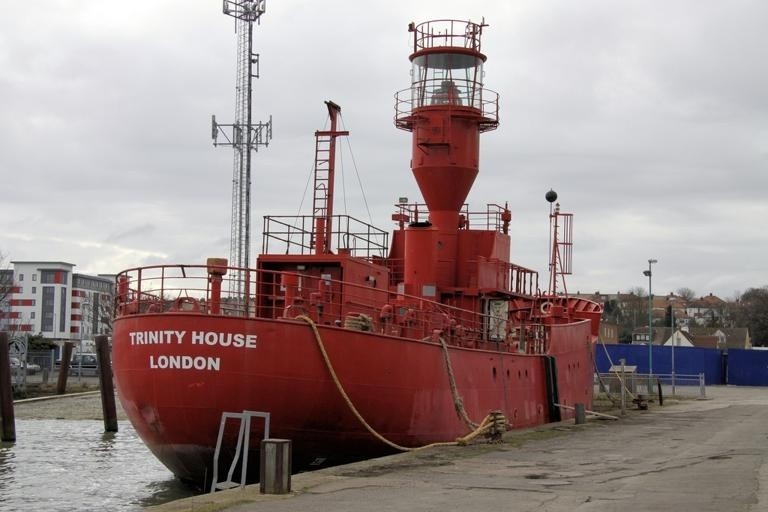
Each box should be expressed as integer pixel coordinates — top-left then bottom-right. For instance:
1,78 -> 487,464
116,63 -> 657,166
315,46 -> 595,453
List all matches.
56,350 -> 100,377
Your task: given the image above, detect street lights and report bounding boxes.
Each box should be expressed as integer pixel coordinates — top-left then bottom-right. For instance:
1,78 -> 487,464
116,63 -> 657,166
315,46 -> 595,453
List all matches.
643,257 -> 658,394
668,292 -> 677,396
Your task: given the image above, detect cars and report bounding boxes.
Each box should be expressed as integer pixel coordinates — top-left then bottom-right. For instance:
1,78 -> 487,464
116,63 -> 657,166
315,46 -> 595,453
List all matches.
9,357 -> 41,376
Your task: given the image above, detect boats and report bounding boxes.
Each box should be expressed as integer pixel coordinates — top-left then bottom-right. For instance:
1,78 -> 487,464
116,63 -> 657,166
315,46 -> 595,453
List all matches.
112,1 -> 606,491
